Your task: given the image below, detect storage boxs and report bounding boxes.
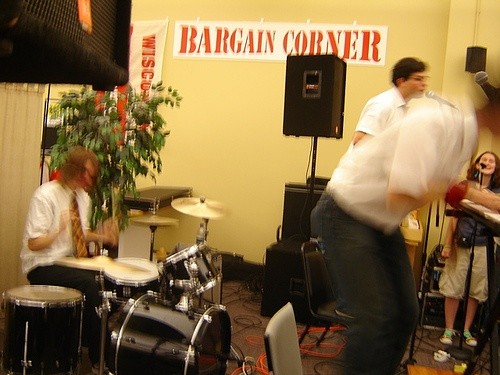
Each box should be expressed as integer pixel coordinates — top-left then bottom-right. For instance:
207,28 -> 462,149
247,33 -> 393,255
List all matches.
398,219 -> 423,242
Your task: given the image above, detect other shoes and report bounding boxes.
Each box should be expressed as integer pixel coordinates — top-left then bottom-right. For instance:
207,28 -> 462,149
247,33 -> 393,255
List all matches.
464,330 -> 477,346
439,329 -> 454,345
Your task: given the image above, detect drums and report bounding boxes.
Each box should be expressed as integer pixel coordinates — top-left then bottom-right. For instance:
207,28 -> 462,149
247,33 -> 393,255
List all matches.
104,256 -> 161,300
105,289 -> 233,375
1,285 -> 83,375
156,244 -> 224,296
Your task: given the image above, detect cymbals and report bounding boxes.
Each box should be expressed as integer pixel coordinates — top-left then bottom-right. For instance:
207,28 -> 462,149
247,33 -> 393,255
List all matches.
170,197 -> 231,219
128,216 -> 181,226
54,256 -> 153,277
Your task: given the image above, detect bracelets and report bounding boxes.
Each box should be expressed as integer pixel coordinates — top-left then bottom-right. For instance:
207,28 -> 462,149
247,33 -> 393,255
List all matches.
54,227 -> 60,234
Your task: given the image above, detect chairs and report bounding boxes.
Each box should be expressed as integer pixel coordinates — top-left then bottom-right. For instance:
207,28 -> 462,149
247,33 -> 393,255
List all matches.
264,301 -> 302,375
299,242 -> 351,348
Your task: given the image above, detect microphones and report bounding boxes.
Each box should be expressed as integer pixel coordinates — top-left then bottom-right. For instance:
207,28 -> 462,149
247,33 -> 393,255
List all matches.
476,71 -> 497,100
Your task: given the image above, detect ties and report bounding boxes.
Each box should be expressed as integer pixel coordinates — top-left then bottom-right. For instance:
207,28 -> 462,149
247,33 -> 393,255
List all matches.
70,191 -> 87,257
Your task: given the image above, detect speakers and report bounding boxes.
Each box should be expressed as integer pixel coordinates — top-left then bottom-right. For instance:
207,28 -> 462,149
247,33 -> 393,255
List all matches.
0,0 -> 132,91
283,54 -> 347,139
260,182 -> 336,328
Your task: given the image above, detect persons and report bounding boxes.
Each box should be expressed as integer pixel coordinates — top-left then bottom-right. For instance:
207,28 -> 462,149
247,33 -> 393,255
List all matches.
309,56 -> 500,375
19,144 -> 117,375
438,151 -> 500,347
